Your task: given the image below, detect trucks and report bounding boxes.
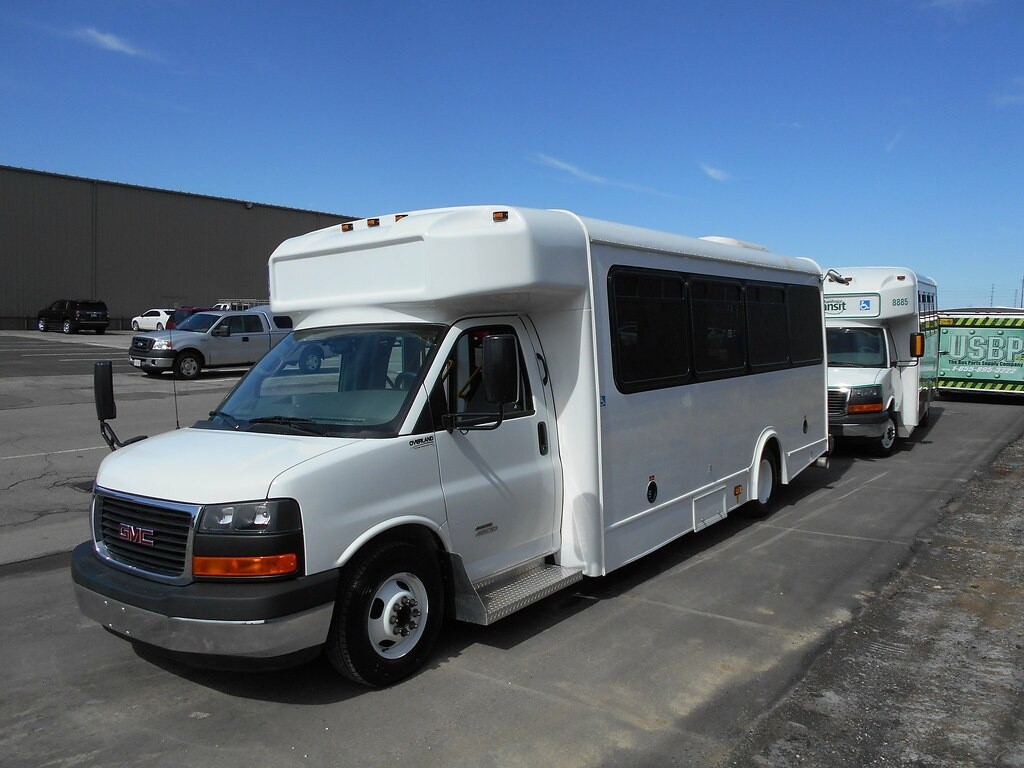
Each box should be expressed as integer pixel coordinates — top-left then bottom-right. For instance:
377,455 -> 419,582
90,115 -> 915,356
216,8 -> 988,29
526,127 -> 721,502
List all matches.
817,266 -> 941,458
69,202 -> 832,691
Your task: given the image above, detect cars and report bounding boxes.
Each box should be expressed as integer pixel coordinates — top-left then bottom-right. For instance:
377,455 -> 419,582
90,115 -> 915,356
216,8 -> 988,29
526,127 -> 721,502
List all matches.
131,308 -> 175,331
165,306 -> 257,331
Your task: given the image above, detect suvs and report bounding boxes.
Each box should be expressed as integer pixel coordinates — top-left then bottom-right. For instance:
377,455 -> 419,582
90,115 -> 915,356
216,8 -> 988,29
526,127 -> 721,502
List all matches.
37,299 -> 110,335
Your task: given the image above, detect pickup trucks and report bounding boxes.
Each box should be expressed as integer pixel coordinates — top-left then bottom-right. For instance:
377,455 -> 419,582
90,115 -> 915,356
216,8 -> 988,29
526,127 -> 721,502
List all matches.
128,305 -> 339,381
